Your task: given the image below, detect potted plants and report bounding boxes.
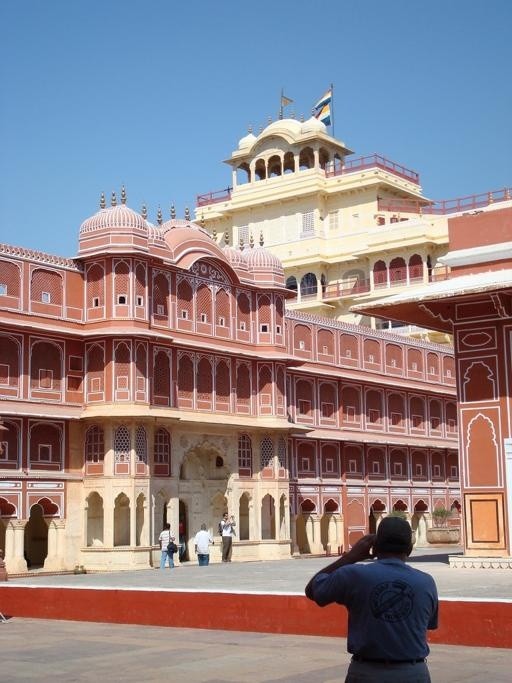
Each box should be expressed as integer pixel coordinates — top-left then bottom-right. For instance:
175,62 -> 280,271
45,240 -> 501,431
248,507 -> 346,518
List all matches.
386,509 -> 415,548
426,507 -> 459,543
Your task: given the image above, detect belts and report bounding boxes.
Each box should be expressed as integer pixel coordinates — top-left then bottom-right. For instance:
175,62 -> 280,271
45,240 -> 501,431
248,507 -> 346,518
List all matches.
353,657 -> 424,664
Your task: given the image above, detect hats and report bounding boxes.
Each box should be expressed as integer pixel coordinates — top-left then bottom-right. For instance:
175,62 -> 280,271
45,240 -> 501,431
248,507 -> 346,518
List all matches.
376,517 -> 412,552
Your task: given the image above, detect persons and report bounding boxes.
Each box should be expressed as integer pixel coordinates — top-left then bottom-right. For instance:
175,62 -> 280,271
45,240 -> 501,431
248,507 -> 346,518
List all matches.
193,523 -> 215,567
158,523 -> 175,570
220,512 -> 237,562
179,513 -> 187,562
303,516 -> 441,683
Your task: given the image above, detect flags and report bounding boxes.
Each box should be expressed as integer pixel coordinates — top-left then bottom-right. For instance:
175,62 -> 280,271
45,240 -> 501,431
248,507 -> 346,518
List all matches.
315,102 -> 332,126
314,88 -> 332,109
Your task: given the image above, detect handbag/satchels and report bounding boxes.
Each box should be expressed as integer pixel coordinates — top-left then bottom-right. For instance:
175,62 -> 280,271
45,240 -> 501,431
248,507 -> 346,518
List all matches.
167,542 -> 177,552
